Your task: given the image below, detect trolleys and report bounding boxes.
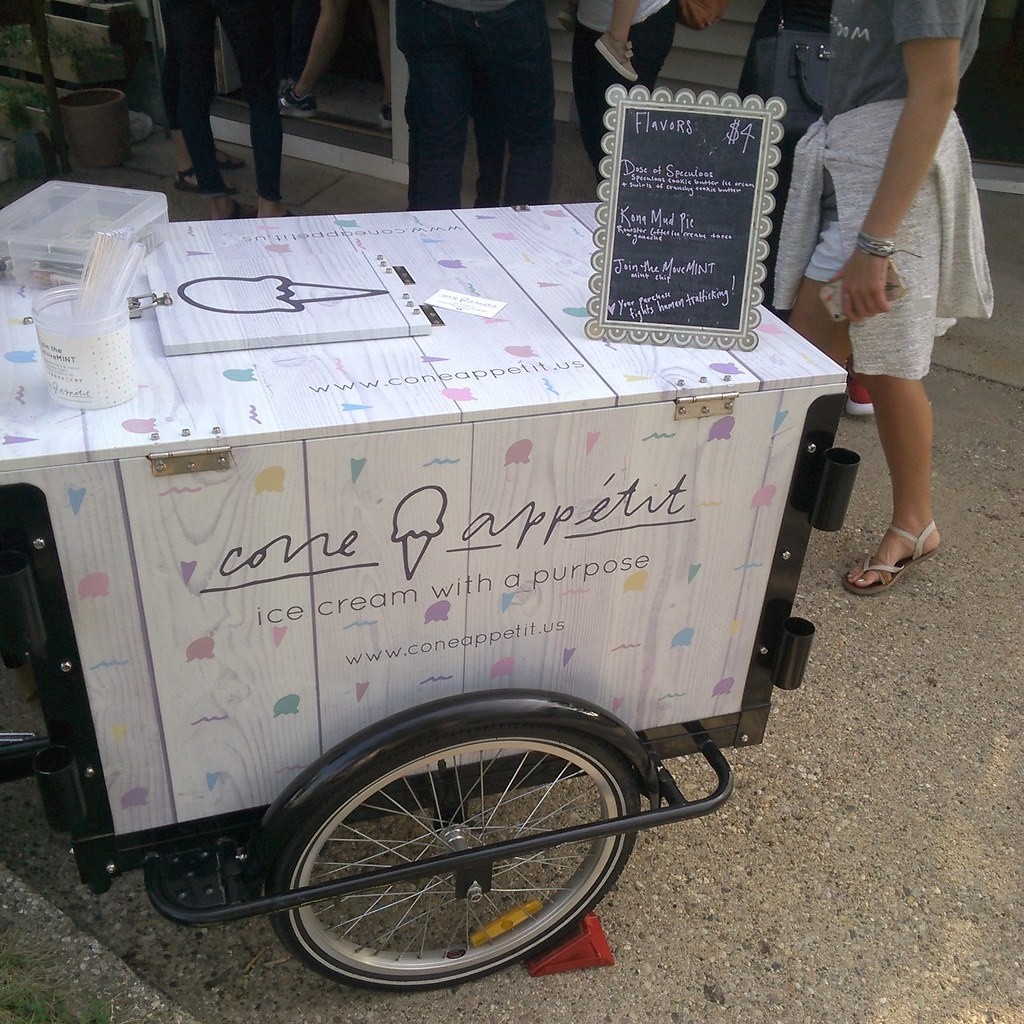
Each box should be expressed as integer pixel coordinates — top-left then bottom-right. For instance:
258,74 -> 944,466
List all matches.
0,201 -> 864,990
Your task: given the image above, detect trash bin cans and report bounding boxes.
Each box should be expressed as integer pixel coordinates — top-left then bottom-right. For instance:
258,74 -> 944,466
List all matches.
56,87 -> 134,171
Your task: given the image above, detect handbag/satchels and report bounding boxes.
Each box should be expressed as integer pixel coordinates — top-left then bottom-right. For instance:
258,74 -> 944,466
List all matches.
756,30 -> 829,131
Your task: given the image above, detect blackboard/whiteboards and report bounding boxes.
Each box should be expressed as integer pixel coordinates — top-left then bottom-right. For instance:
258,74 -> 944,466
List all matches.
582,82 -> 788,353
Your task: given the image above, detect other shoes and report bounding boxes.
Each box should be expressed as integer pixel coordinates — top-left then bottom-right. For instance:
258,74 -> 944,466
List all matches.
176,166 -> 225,193
228,200 -> 241,218
284,210 -> 291,215
214,147 -> 245,169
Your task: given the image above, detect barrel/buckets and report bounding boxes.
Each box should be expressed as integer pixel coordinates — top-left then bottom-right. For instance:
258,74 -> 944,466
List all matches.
60,88 -> 128,166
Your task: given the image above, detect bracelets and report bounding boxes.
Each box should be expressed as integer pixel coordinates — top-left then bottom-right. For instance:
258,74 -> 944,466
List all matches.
856,231 -> 895,257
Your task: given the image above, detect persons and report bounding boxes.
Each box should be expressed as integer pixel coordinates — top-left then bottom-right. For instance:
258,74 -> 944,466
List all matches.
160,0 -> 296,220
281,0 -> 392,128
731,0 -> 995,595
394,0 -> 557,211
572,0 -> 676,185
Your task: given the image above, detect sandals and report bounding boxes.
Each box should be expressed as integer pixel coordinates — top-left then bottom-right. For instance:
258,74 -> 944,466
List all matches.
843,517 -> 939,594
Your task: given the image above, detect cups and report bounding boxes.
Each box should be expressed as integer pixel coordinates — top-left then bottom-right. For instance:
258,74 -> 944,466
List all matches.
31,286 -> 135,410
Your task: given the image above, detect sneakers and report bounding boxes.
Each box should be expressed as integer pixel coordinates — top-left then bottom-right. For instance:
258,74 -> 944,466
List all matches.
278,84 -> 317,118
558,7 -> 576,32
846,380 -> 874,416
379,101 -> 392,129
594,30 -> 638,82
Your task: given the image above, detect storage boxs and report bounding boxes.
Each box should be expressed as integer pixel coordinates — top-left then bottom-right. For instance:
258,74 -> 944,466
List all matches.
0,180 -> 170,291
